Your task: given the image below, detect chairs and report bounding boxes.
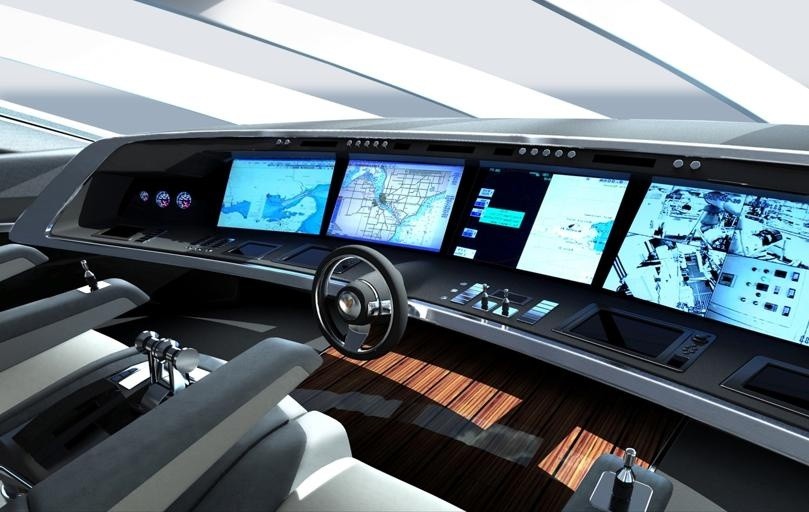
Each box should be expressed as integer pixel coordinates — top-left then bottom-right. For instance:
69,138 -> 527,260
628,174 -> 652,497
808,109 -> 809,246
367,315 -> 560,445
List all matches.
0,244 -> 149,411
0,339 -> 674,512
